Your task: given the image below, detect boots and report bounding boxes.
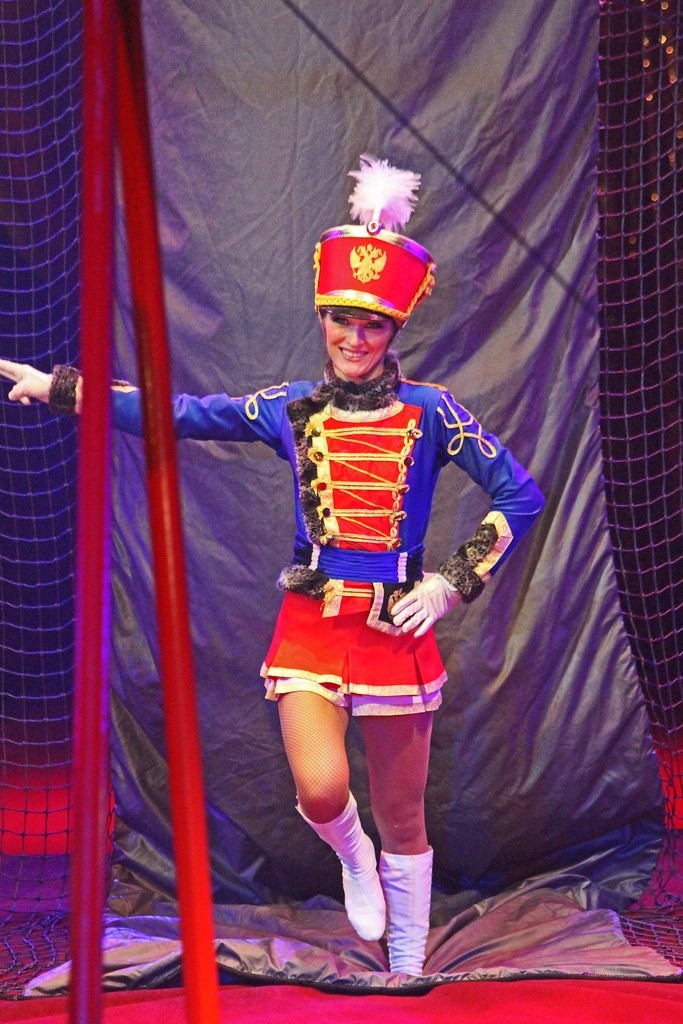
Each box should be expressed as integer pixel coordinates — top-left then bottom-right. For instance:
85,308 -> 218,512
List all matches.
379,845 -> 433,976
295,791 -> 386,941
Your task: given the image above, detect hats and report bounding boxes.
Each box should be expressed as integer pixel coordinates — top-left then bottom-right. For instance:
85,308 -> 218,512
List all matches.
312,154 -> 437,330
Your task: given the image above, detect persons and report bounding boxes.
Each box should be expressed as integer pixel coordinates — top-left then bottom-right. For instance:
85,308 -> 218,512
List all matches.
0,151 -> 547,977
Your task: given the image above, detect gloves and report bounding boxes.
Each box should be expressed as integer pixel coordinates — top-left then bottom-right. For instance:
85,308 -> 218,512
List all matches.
391,570 -> 463,638
0,359 -> 53,406
